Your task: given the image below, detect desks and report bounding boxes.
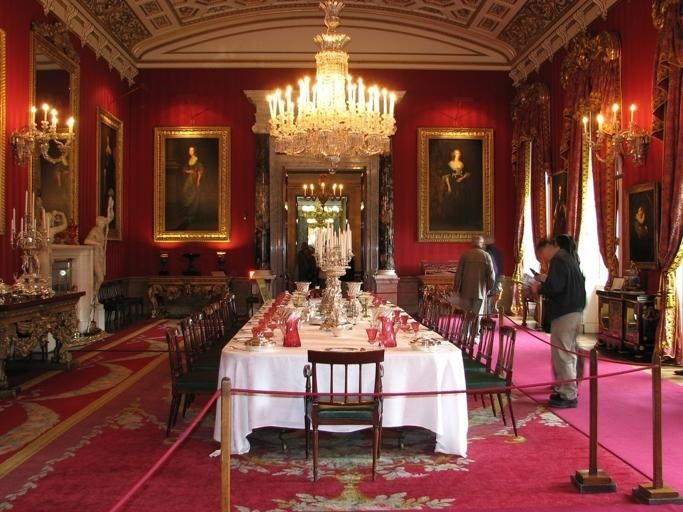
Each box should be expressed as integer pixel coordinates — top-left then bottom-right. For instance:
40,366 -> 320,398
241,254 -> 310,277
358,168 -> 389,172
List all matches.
0,291 -> 87,400
146,275 -> 236,319
415,273 -> 503,320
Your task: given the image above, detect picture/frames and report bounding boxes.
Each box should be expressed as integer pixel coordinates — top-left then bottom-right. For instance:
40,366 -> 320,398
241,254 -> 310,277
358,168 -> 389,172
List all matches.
623,180 -> 661,272
417,126 -> 495,244
96,105 -> 125,242
549,168 -> 568,241
153,125 -> 232,243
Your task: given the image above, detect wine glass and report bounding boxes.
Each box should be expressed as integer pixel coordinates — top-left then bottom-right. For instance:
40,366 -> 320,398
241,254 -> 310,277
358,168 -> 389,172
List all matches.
400,315 -> 408,329
260,329 -> 276,346
266,320 -> 278,338
393,309 -> 401,324
410,322 -> 419,341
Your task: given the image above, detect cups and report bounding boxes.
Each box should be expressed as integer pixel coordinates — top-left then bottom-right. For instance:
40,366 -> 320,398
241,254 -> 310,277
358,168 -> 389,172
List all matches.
365,328 -> 377,343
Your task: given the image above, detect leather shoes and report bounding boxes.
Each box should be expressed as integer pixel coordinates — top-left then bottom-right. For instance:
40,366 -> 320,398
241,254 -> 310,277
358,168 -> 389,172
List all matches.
549,394 -> 577,403
549,401 -> 575,408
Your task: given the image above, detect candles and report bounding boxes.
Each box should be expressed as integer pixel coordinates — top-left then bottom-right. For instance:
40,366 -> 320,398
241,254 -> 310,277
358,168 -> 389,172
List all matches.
582,103 -> 637,133
301,180 -> 343,196
10,189 -> 50,243
315,222 -> 353,261
28,101 -> 75,134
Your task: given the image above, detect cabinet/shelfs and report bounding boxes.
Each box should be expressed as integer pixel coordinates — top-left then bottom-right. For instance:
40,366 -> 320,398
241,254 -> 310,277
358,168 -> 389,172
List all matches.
594,290 -> 658,359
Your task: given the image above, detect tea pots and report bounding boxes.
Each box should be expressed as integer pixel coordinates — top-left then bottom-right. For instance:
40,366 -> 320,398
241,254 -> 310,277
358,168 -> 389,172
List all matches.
378,315 -> 402,348
279,317 -> 302,347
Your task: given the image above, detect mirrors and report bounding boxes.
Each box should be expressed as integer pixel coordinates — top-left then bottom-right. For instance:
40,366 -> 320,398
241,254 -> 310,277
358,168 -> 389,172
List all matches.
23,14 -> 82,248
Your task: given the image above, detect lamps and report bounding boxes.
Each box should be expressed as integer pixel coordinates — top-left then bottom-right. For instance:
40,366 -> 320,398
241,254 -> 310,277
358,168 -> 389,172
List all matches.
215,251 -> 226,271
263,0 -> 400,177
158,253 -> 169,276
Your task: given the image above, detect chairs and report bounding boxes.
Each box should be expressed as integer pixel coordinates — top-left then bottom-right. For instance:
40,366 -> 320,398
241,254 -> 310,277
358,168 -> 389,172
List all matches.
99,279 -> 144,332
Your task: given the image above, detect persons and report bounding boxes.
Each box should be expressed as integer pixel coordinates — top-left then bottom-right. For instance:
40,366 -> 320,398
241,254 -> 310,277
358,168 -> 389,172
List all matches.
82,214 -> 109,304
451,234 -> 497,345
36,210 -> 69,285
180,143 -> 205,218
444,148 -> 470,224
526,238 -> 586,409
632,201 -> 656,259
295,242 -> 316,281
552,179 -> 568,230
100,131 -> 116,217
550,232 -> 582,392
484,242 -> 504,318
